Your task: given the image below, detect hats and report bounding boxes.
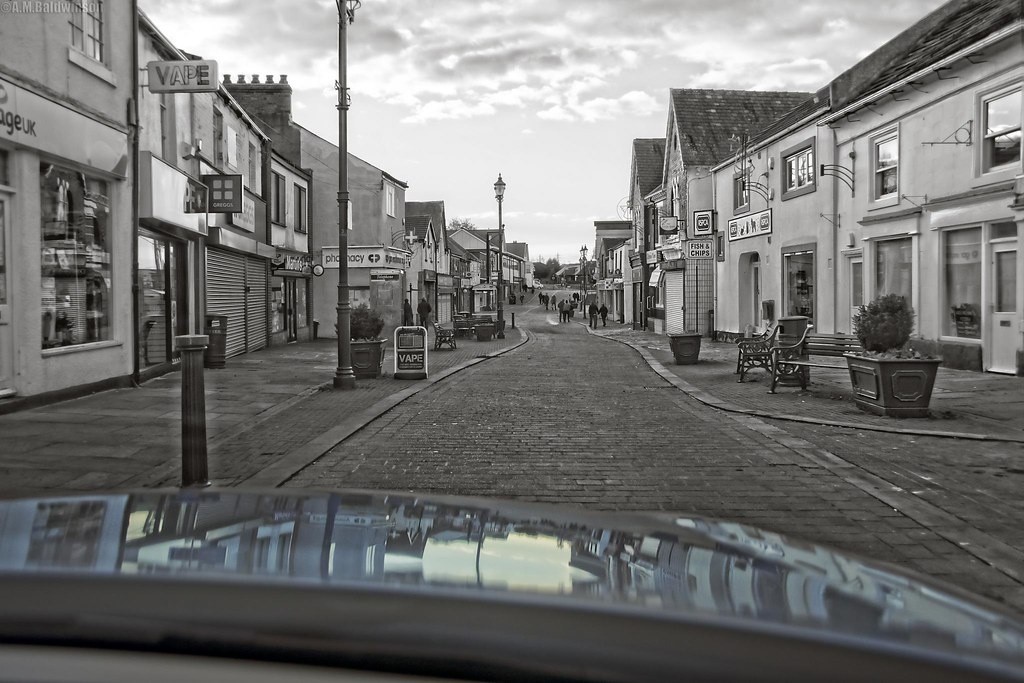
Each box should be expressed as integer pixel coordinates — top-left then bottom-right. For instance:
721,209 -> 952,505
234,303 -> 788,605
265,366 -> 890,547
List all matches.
404,299 -> 409,301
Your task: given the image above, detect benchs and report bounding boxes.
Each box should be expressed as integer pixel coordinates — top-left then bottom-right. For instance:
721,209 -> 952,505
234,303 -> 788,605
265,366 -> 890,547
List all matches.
767,326 -> 865,394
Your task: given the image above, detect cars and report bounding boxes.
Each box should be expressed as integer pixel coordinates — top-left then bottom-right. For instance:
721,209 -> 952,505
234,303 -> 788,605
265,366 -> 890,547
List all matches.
533,281 -> 543,289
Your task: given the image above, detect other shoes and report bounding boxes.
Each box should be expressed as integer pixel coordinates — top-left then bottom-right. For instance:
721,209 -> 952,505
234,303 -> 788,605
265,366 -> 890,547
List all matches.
603,324 -> 606,327
589,325 -> 592,327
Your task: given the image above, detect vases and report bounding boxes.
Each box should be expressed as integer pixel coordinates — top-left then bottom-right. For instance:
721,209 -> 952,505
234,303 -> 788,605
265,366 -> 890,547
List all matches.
667,332 -> 703,365
472,323 -> 496,342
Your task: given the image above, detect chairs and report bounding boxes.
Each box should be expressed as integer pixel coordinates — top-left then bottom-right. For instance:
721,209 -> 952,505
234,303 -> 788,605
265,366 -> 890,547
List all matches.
429,318 -> 456,351
453,315 -> 492,340
734,321 -> 784,383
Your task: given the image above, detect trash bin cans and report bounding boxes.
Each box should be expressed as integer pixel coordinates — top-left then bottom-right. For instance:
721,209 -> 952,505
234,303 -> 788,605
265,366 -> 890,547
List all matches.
204,312 -> 229,369
777,316 -> 812,386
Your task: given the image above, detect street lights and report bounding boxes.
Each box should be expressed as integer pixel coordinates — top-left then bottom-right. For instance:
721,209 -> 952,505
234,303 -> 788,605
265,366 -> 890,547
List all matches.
494,173 -> 506,339
580,244 -> 588,318
335,0 -> 361,388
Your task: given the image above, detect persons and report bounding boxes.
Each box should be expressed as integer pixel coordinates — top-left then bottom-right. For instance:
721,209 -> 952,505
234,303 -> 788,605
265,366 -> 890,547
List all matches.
538,292 -> 549,310
522,282 -> 528,292
557,299 -> 570,322
519,294 -> 525,304
588,300 -> 599,329
403,299 -> 412,325
598,303 -> 608,326
568,291 -> 580,303
550,295 -> 557,310
417,297 -> 432,331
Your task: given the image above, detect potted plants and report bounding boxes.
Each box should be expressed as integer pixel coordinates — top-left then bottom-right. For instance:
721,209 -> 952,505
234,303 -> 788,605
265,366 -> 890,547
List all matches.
334,302 -> 389,379
843,293 -> 945,418
